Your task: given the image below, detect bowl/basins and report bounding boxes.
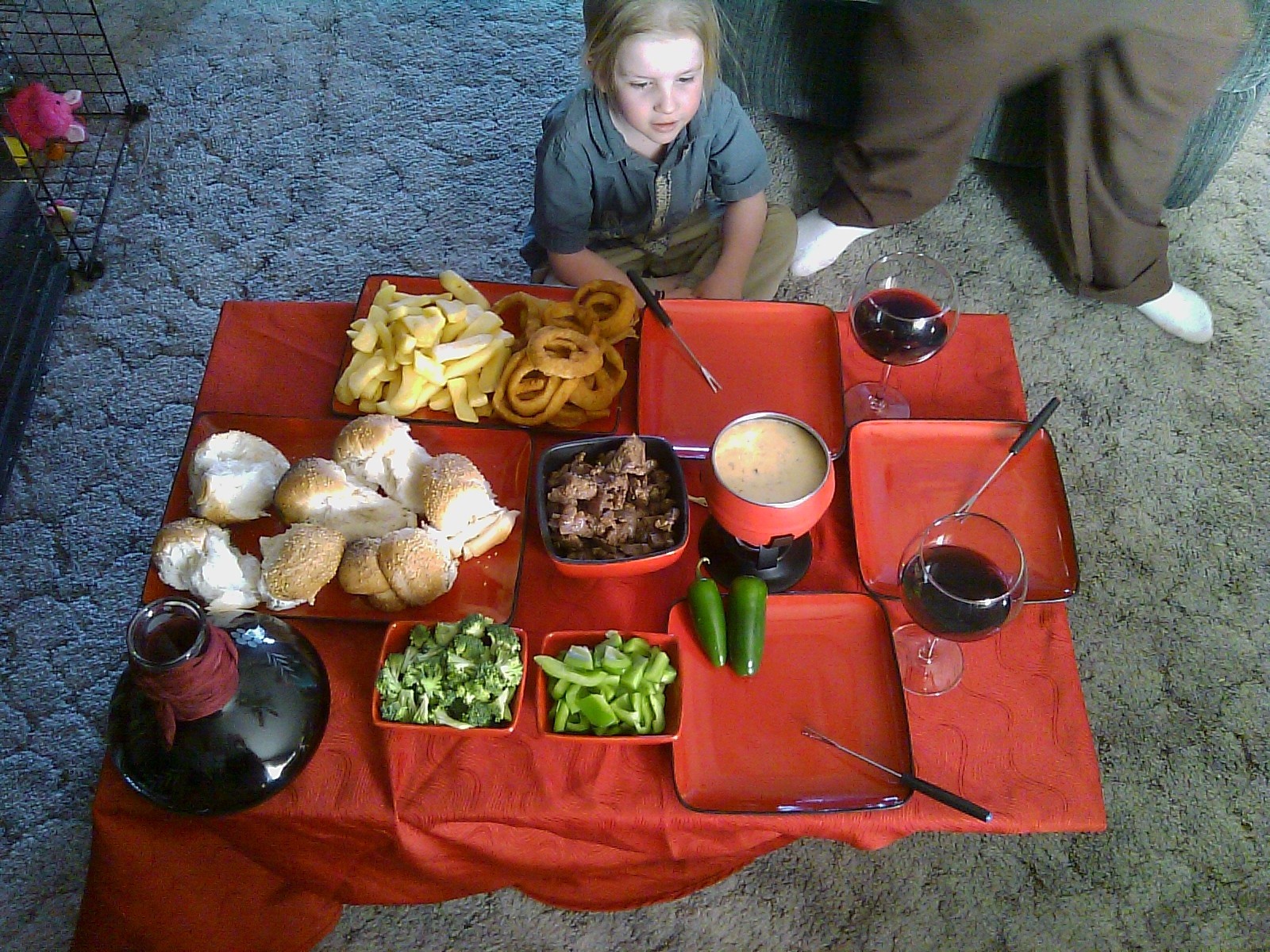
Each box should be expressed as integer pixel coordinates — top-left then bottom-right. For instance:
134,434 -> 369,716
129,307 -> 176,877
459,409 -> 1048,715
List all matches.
372,618 -> 529,738
534,434 -> 691,579
533,629 -> 687,746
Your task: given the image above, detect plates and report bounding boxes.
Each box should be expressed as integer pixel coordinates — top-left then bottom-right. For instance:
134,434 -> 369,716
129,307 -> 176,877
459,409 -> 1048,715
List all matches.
849,422 -> 1082,605
138,411 -> 535,630
330,273 -> 630,436
635,298 -> 848,465
666,589 -> 916,814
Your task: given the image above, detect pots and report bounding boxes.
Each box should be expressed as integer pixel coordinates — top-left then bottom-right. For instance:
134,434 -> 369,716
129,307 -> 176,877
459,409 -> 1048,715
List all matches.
701,416 -> 837,546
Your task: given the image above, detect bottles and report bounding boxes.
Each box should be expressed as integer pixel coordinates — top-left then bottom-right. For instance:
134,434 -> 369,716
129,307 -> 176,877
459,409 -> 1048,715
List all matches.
106,596 -> 333,816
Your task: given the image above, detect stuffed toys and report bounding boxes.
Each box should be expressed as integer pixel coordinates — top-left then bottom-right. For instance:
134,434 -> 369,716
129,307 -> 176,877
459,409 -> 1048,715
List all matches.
0,82 -> 89,149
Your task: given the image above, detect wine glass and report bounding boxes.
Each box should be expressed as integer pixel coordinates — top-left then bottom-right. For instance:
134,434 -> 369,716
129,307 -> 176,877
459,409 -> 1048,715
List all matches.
846,252 -> 960,431
892,514 -> 1030,697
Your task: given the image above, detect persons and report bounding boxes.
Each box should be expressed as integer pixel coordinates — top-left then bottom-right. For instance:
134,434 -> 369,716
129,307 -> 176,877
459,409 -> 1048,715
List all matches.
515,0 -> 798,302
788,0 -> 1244,346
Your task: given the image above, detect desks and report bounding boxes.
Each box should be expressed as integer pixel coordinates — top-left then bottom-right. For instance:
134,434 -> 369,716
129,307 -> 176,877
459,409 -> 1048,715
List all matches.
70,301 -> 1110,952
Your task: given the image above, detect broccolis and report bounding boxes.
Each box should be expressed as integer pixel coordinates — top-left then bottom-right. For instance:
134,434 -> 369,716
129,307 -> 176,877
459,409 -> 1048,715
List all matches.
374,611 -> 524,730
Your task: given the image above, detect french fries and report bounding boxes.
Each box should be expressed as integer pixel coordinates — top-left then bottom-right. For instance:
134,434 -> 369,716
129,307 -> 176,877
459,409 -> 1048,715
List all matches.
336,269 -> 515,423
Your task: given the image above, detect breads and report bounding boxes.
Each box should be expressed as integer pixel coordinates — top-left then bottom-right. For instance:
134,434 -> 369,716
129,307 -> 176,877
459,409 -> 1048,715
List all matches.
151,413 -> 521,614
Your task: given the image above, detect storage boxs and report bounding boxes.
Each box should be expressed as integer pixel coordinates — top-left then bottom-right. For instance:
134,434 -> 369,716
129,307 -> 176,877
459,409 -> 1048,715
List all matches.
0,177 -> 73,500
0,0 -> 151,283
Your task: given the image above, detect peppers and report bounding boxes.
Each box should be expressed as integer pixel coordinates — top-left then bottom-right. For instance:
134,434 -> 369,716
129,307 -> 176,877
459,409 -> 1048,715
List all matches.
688,557 -> 728,667
532,630 -> 678,737
728,576 -> 768,676
